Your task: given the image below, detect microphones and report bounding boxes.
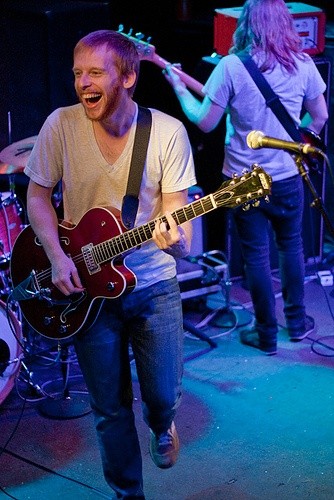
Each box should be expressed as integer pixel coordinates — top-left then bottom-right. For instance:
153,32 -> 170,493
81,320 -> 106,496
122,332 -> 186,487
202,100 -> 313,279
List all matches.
246,130 -> 322,155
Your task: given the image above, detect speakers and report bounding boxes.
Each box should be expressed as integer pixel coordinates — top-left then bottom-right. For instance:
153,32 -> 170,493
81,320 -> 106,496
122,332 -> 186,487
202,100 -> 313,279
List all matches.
226,156 -> 324,284
174,186 -> 208,282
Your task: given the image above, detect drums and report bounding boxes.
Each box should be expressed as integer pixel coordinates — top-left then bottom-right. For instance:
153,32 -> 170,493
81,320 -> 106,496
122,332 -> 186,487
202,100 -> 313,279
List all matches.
0,299 -> 24,404
0,191 -> 28,271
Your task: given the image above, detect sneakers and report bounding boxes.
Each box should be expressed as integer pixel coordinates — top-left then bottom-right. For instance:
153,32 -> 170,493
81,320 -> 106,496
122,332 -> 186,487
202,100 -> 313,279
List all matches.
142,412 -> 179,468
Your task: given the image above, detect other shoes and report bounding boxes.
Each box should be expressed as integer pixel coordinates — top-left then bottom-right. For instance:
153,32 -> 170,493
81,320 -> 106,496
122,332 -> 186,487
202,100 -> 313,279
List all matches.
289,314 -> 316,340
239,329 -> 279,356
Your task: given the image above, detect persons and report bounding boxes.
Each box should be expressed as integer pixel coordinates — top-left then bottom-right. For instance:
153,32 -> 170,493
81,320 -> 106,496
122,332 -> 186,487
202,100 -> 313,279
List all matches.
24,30 -> 198,500
162,0 -> 329,356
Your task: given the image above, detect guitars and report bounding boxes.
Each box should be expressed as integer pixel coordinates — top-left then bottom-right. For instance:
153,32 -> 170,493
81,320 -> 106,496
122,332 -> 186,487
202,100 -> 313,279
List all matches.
10,163 -> 273,341
115,23 -> 328,166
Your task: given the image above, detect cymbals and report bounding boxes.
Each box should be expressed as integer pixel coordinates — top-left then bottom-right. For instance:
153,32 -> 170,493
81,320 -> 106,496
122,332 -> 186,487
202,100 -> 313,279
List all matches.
0,159 -> 25,175
0,135 -> 40,167
